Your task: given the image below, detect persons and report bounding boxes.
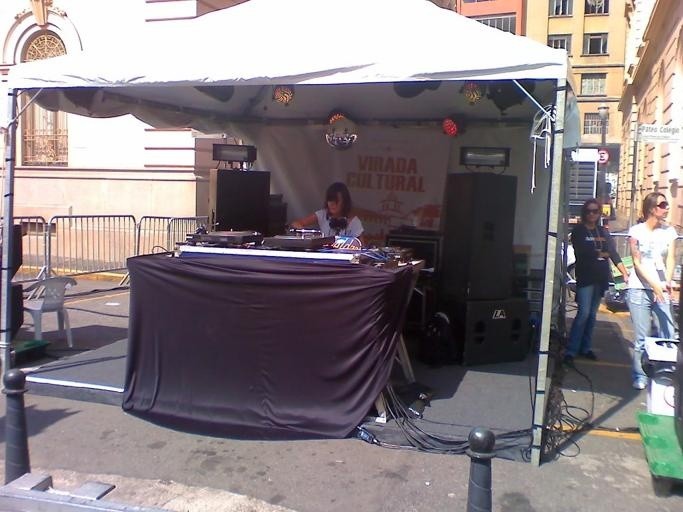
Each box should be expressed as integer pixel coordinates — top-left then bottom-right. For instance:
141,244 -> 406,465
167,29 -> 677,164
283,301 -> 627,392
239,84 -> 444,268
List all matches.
563,197 -> 630,366
292,181 -> 368,248
626,192 -> 678,389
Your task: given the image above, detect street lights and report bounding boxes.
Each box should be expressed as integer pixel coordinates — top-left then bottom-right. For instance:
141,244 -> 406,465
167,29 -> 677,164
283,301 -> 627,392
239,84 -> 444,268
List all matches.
596,101 -> 609,207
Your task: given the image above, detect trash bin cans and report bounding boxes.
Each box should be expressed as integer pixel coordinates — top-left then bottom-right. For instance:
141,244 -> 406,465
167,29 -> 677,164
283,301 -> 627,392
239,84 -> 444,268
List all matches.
642,336 -> 681,417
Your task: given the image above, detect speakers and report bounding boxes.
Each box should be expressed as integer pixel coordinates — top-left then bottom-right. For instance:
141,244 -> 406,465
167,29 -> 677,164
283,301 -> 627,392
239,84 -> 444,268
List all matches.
440,171 -> 518,301
425,291 -> 531,365
207,168 -> 271,231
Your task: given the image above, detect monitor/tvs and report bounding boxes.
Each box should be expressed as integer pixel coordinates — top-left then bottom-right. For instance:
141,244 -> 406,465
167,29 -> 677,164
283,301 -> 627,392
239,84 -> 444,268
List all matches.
387,229 -> 444,276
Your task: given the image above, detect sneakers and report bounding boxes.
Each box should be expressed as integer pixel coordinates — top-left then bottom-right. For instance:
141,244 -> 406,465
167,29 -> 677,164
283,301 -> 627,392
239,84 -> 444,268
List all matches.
579,351 -> 596,359
633,379 -> 645,389
564,355 -> 573,364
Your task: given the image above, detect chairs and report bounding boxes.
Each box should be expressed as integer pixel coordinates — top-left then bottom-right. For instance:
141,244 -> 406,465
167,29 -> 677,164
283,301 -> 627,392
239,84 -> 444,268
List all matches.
22,277 -> 78,348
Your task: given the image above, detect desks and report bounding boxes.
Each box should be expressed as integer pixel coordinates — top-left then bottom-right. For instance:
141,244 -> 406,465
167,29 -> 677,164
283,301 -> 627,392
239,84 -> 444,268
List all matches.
121,250 -> 425,441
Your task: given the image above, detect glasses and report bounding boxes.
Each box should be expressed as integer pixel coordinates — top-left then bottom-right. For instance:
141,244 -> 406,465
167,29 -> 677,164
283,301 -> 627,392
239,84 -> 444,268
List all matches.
584,209 -> 599,215
657,201 -> 668,209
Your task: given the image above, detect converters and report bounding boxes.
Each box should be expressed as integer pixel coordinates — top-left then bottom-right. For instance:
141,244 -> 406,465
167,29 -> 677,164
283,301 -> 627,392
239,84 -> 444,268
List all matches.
358,427 -> 377,445
